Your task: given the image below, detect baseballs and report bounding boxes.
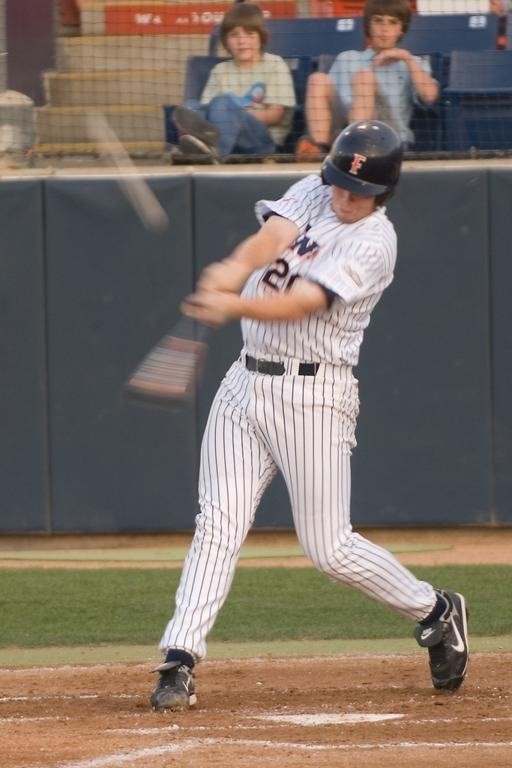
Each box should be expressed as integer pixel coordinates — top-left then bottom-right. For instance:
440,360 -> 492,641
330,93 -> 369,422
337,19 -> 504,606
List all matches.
89,111 -> 168,233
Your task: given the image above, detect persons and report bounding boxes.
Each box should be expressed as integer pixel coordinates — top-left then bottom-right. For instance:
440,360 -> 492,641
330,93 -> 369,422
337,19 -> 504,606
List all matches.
292,0 -> 441,163
148,119 -> 469,714
169,2 -> 298,167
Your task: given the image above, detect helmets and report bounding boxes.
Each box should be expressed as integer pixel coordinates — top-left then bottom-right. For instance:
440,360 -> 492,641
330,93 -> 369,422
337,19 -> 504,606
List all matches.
323,119 -> 403,195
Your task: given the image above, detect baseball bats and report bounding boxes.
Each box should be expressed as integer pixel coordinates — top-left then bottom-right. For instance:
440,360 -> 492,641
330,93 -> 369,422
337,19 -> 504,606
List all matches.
125,315 -> 209,417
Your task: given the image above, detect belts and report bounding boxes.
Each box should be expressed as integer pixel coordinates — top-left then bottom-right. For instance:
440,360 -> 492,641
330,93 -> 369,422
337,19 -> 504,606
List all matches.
238,354 -> 321,377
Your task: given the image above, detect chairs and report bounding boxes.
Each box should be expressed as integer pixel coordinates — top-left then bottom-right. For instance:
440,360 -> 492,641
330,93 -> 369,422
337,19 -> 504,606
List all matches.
161,8 -> 511,160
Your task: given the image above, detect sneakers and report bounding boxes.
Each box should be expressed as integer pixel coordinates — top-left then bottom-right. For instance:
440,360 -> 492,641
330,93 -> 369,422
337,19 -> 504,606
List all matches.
414,590 -> 468,692
148,661 -> 198,711
174,106 -> 218,164
